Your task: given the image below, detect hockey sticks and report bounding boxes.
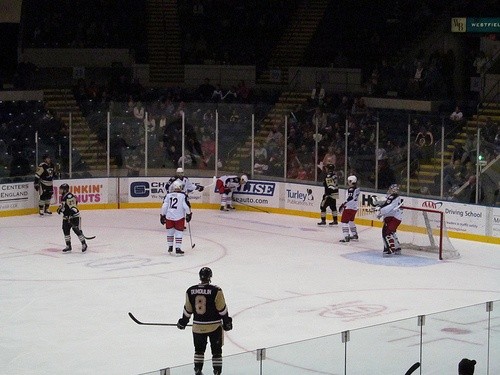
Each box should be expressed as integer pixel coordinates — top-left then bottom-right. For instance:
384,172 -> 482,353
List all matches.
233,198 -> 269,213
63,212 -> 96,240
189,222 -> 195,248
129,312 -> 192,326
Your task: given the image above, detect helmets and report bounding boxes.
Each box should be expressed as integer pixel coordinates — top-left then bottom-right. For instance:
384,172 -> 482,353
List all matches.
200,267 -> 212,280
241,174 -> 248,182
59,183 -> 69,191
348,176 -> 357,183
177,168 -> 184,172
325,162 -> 334,170
42,153 -> 50,160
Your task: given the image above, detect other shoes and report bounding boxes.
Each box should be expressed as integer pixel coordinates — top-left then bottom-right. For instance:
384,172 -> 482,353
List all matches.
382,241 -> 401,255
350,233 -> 358,240
227,204 -> 235,210
81,240 -> 88,252
168,246 -> 184,256
62,243 -> 72,253
45,209 -> 52,215
39,210 -> 44,216
339,234 -> 350,243
194,367 -> 223,375
318,221 -> 326,226
329,221 -> 338,226
220,206 -> 229,212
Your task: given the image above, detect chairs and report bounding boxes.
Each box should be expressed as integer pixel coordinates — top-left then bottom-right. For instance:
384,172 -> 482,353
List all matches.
73,81 -> 483,200
0,98 -> 93,183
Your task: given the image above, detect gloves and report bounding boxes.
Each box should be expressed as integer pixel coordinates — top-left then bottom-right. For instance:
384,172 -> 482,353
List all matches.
161,215 -> 166,225
376,211 -> 383,220
177,317 -> 189,329
195,185 -> 204,192
186,213 -> 193,222
34,184 -> 40,192
339,204 -> 345,213
224,187 -> 231,195
222,317 -> 233,331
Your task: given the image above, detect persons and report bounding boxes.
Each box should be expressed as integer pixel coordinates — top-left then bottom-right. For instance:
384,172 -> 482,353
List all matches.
57,183 -> 87,253
458,358 -> 476,375
317,163 -> 339,226
176,266 -> 233,375
376,183 -> 405,256
216,174 -> 248,211
34,154 -> 62,215
164,167 -> 204,229
338,175 -> 361,243
0,39 -> 500,207
159,180 -> 192,254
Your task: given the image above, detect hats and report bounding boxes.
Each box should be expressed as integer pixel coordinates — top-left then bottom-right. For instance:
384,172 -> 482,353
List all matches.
389,183 -> 399,194
